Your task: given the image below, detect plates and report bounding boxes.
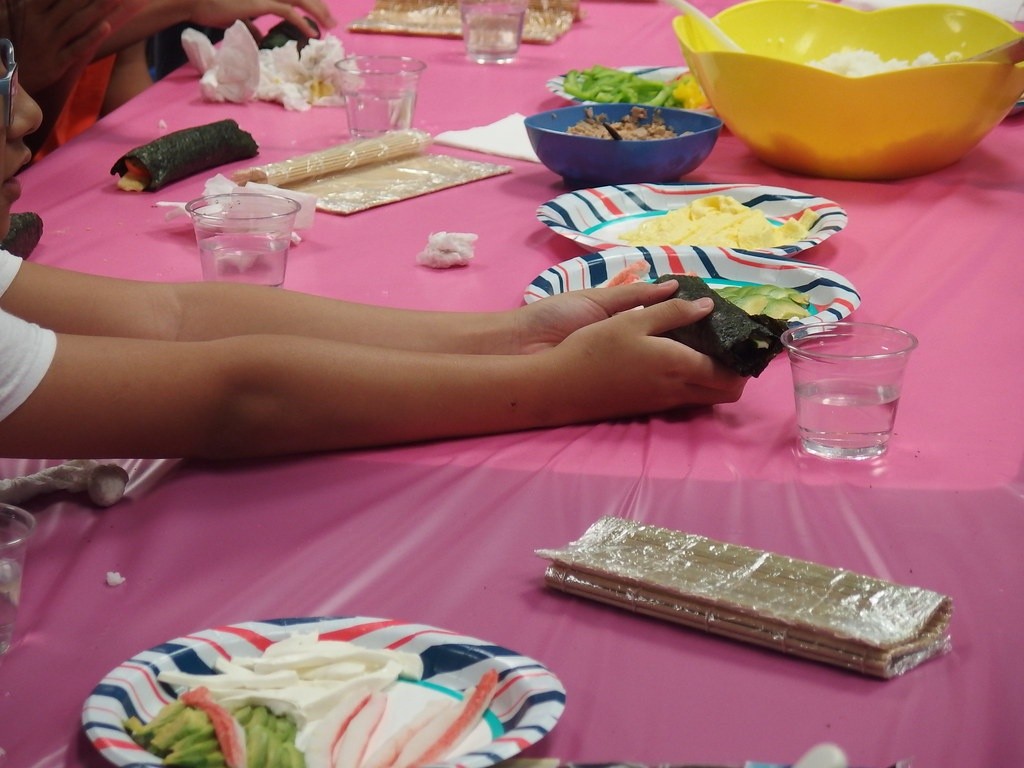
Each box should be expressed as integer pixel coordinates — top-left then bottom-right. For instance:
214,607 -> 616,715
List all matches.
546,65 -> 718,119
523,245 -> 862,322
535,181 -> 849,256
82,616 -> 569,768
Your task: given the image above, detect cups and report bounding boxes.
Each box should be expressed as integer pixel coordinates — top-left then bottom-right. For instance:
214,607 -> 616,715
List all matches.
457,0 -> 530,65
780,324 -> 919,462
185,193 -> 302,289
333,55 -> 427,140
0,503 -> 37,657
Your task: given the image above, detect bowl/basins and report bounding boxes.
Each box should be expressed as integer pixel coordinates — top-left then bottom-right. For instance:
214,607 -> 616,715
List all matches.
671,0 -> 1024,181
523,103 -> 724,189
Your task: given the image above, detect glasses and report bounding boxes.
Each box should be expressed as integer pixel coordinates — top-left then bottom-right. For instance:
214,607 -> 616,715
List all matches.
0,38 -> 18,126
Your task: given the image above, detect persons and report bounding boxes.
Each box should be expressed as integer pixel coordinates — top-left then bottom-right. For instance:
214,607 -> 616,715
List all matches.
1,0 -> 150,174
0,55 -> 752,460
29,1 -> 337,168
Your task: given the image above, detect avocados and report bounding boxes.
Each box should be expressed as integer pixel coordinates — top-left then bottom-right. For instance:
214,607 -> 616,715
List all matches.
710,284 -> 810,320
125,692 -> 304,768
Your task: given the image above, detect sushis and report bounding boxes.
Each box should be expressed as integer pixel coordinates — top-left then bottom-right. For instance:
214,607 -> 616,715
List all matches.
106,117 -> 260,192
642,272 -> 792,379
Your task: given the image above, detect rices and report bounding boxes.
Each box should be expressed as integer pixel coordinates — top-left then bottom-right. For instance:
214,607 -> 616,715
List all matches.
802,44 -> 962,78
